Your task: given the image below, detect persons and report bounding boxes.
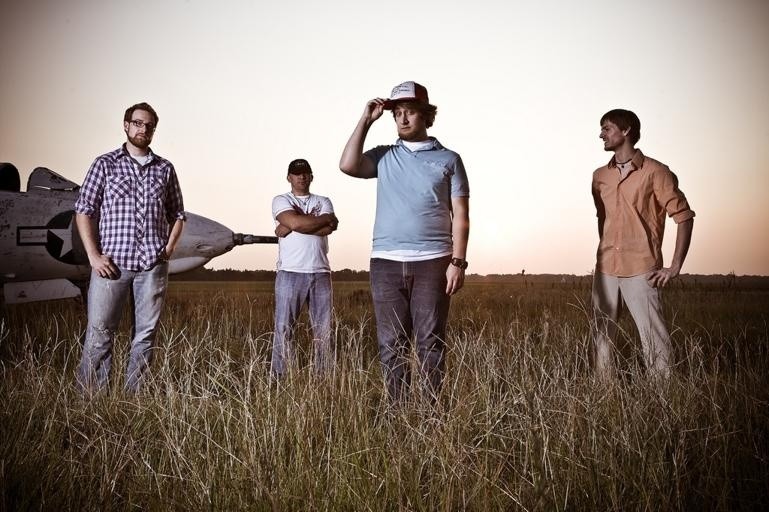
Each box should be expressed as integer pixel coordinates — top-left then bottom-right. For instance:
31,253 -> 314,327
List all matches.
271,158 -> 339,378
589,107 -> 696,385
74,103 -> 186,398
339,81 -> 472,400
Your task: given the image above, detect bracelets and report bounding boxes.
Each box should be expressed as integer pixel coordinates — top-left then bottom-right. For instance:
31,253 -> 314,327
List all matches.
448,258 -> 468,270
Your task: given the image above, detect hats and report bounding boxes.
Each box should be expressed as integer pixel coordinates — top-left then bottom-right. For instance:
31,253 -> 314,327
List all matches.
288,159 -> 312,175
382,81 -> 430,110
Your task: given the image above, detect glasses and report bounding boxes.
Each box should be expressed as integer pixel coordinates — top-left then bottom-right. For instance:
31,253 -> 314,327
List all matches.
129,119 -> 155,130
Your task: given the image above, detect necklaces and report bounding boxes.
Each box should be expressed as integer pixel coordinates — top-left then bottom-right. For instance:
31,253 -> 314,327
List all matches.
615,158 -> 633,169
291,190 -> 311,205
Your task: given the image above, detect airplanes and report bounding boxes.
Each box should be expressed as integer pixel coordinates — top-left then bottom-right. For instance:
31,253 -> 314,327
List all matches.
0,163 -> 277,308
